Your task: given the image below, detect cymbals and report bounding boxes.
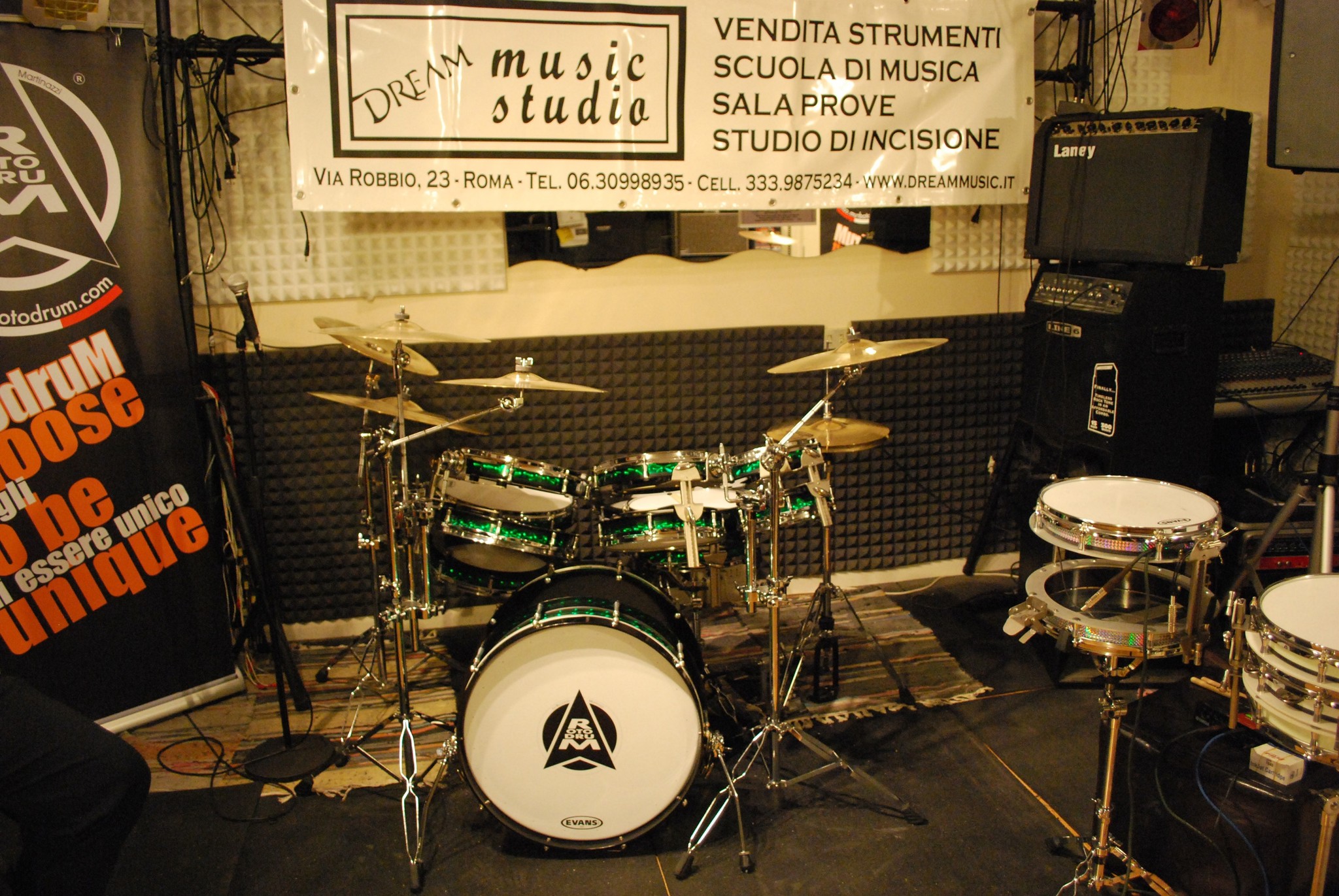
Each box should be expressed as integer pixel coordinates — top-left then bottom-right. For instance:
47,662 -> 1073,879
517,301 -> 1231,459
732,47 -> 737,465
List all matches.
739,230 -> 795,245
767,418 -> 890,453
308,392 -> 490,436
309,319 -> 492,343
315,316 -> 439,376
767,332 -> 949,374
435,372 -> 606,394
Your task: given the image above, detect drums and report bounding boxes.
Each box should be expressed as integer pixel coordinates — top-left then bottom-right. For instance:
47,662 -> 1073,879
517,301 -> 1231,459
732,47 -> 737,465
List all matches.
729,439 -> 835,533
456,560 -> 711,851
593,450 -> 747,552
1024,476 -> 1222,659
1241,573 -> 1339,769
432,447 -> 594,560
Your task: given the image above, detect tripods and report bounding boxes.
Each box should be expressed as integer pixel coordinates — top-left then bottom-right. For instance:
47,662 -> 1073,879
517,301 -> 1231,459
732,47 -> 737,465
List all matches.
313,349 -> 525,891
671,363 -> 930,883
1046,545 -> 1187,896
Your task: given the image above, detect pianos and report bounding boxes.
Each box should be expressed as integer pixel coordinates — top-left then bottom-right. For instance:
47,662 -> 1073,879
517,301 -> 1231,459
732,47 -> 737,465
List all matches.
1213,347 -> 1333,418
